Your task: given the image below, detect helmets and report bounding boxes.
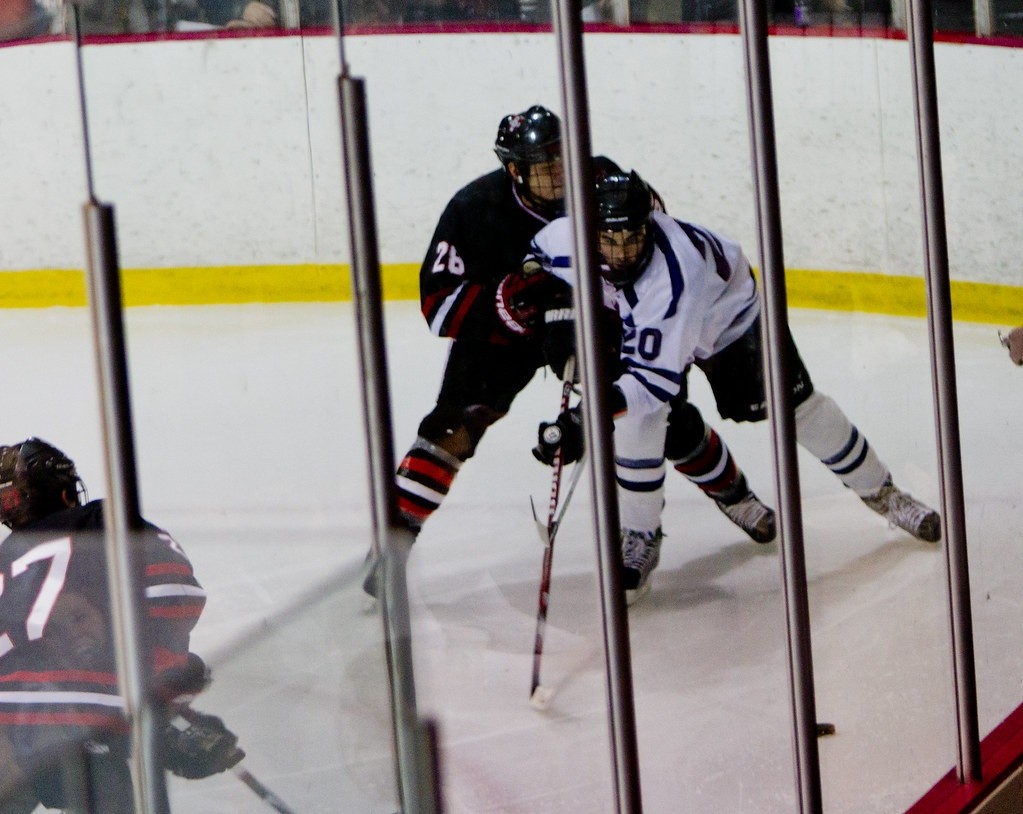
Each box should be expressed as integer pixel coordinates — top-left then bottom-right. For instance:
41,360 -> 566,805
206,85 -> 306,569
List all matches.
594,170 -> 654,286
492,105 -> 562,224
0,436 -> 90,528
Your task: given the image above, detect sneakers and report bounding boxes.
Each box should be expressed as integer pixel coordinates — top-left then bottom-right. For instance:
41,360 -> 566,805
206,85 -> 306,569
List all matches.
618,529 -> 668,606
361,528 -> 415,614
863,478 -> 942,548
714,484 -> 777,547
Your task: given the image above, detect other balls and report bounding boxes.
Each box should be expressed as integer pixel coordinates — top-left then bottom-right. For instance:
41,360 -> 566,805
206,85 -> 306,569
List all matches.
816,722 -> 836,737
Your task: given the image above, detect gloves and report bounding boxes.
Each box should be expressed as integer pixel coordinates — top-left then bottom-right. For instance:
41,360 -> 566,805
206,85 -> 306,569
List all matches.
496,270 -> 551,334
162,652 -> 212,705
531,407 -> 586,466
162,709 -> 246,779
546,293 -> 584,384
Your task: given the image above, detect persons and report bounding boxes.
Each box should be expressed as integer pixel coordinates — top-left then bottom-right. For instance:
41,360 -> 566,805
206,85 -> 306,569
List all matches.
523,172 -> 944,604
0,439 -> 246,814
0,0 -> 552,41
356,106 -> 779,595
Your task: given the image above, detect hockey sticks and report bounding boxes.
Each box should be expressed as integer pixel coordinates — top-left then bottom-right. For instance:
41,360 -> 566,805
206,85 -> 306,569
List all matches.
528,447 -> 587,549
528,353 -> 576,714
228,762 -> 298,814
